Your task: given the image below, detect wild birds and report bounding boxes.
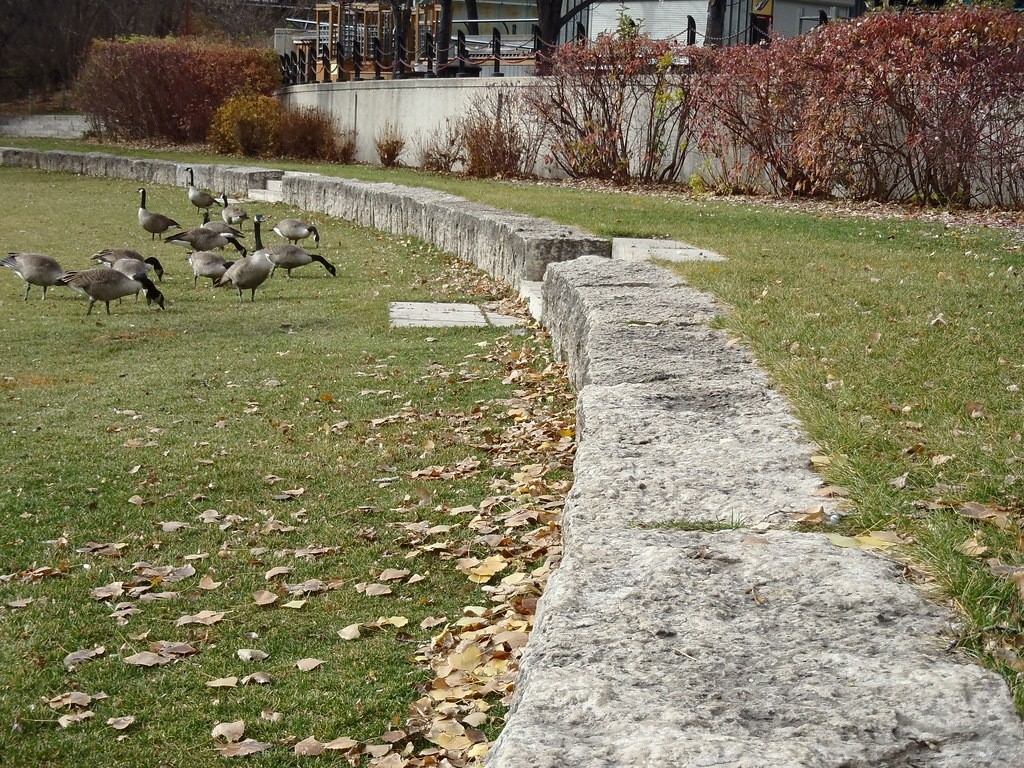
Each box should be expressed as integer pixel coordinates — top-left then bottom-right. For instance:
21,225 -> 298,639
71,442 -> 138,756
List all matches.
56,248 -> 166,316
189,250 -> 234,287
0,252 -> 69,300
183,167 -> 251,231
215,215 -> 336,301
135,188 -> 182,243
164,212 -> 248,258
